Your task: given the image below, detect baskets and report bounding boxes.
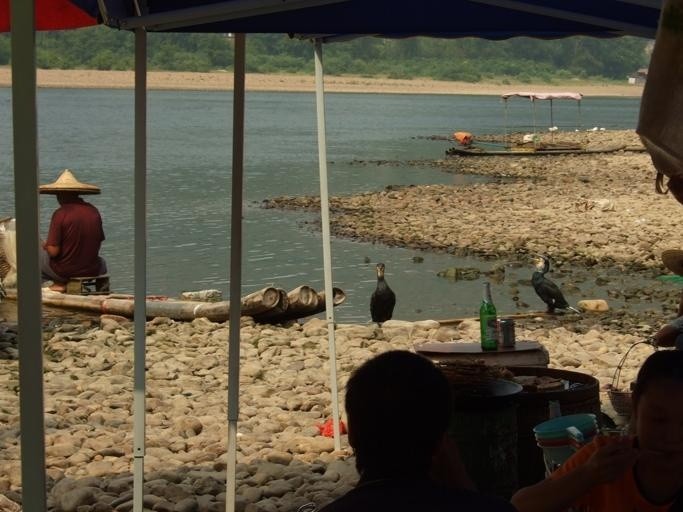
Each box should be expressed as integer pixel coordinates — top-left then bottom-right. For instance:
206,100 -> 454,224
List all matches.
608,338 -> 658,417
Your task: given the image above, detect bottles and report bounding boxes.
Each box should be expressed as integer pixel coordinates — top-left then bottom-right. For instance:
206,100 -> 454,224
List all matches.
479,280 -> 499,350
534,138 -> 540,149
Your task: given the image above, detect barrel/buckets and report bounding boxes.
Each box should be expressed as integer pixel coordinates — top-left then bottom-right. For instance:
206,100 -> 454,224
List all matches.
511,365 -> 601,490
442,374 -> 523,491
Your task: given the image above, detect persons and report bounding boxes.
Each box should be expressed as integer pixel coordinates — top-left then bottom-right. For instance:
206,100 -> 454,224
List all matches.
38,169 -> 106,295
316,350 -> 518,511
510,351 -> 682,511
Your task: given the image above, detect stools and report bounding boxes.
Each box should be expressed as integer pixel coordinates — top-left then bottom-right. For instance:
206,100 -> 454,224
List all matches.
63,272 -> 113,296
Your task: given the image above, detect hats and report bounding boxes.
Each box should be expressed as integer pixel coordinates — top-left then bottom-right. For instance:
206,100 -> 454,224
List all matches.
39,169 -> 102,193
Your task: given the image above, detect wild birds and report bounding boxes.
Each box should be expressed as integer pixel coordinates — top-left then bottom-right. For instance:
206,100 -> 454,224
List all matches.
529,255 -> 581,314
369,262 -> 396,327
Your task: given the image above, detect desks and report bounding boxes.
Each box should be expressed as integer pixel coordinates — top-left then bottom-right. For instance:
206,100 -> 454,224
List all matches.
413,337 -> 549,368
445,365 -> 605,498
